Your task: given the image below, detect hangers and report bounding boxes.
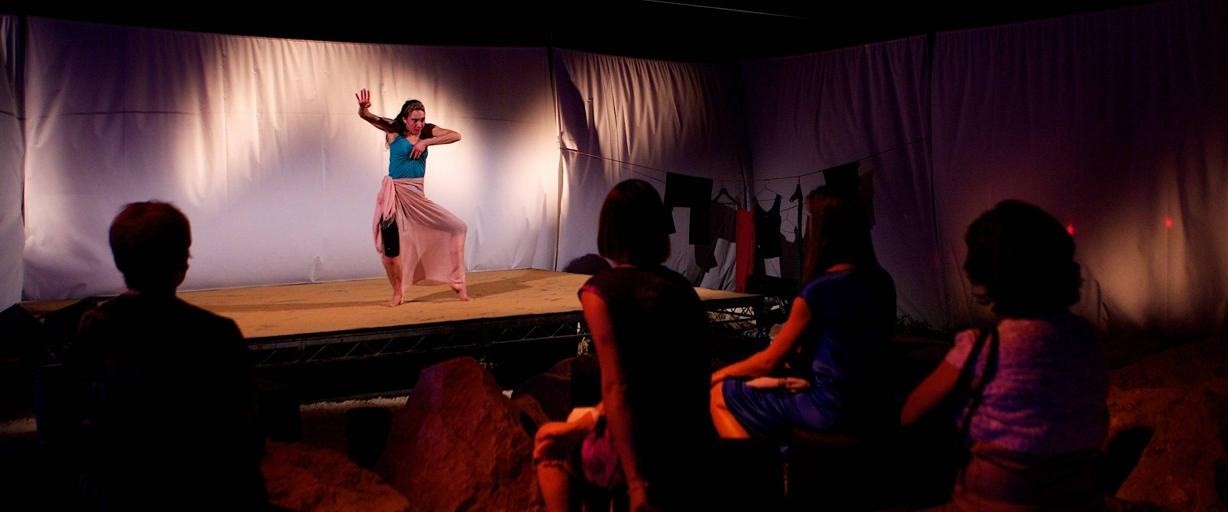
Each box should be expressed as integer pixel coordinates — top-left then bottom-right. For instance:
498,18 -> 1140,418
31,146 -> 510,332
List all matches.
711,176 -> 803,206
779,208 -> 797,234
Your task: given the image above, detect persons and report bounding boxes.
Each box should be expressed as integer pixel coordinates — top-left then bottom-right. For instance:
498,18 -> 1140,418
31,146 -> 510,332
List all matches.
73,201 -> 270,512
532,175 -> 716,511
893,195 -> 1118,511
354,88 -> 475,307
706,179 -> 899,509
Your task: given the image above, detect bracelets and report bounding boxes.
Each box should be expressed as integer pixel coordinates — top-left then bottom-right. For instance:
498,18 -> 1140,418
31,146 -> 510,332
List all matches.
623,477 -> 649,496
